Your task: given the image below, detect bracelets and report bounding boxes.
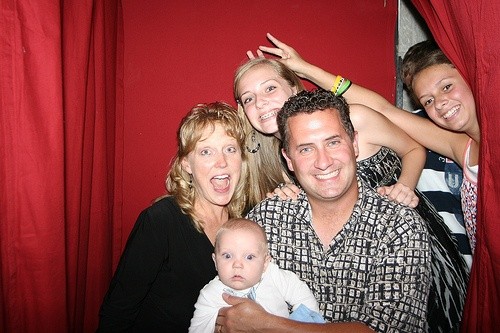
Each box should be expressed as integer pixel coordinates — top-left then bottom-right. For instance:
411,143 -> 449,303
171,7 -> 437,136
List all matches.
330,74 -> 352,97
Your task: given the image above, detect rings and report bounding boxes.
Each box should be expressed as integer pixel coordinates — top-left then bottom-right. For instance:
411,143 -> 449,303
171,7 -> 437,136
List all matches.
217,326 -> 222,333
279,183 -> 286,189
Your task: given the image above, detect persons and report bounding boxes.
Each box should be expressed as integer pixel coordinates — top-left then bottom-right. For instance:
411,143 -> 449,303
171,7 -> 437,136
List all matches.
98,102 -> 244,333
187,218 -> 327,333
213,88 -> 434,333
259,33 -> 481,257
247,39 -> 473,272
232,58 -> 470,333
